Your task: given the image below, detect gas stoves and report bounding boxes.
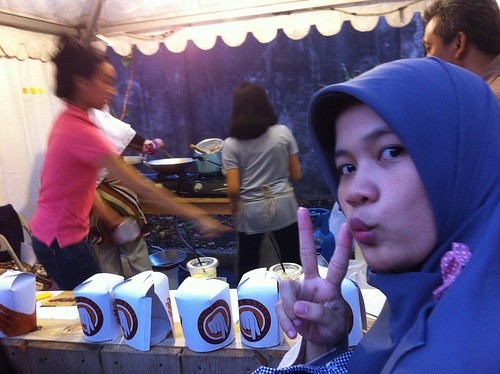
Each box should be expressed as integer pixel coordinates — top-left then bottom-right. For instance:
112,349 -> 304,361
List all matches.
144,177 -> 243,197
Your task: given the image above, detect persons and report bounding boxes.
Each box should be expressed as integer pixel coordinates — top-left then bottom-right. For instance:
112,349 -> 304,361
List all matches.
25,32 -> 233,292
222,82 -> 302,285
275,55 -> 500,374
422,1 -> 500,102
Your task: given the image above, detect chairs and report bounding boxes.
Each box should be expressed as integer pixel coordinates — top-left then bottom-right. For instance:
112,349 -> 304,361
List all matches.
0,203 -> 52,291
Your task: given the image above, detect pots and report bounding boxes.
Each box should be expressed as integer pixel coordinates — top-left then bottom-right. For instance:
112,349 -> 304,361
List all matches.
148,244 -> 187,268
192,138 -> 223,174
122,157 -> 197,174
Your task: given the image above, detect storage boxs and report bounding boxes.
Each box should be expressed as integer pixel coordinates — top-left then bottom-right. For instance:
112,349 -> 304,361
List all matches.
73,273 -> 124,342
299,265 -> 368,348
0,269 -> 37,337
112,271 -> 176,352
236,268 -> 284,348
174,277 -> 236,353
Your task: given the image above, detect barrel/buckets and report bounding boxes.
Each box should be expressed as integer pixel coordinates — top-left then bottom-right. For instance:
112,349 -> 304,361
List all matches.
154,266 -> 179,290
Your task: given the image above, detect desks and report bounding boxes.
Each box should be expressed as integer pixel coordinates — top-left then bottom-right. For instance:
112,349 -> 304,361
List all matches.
0,289 -> 380,374
138,198 -> 233,216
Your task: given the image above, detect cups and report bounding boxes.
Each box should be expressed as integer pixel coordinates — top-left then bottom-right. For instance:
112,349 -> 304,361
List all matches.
187,258 -> 219,279
271,263 -> 302,281
